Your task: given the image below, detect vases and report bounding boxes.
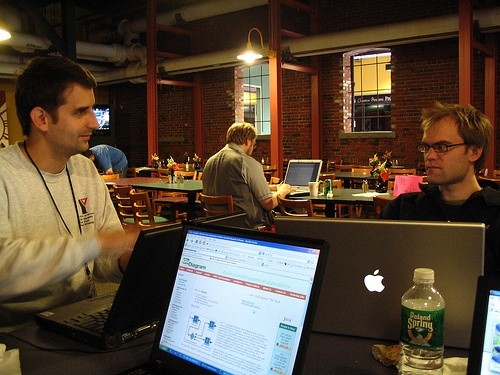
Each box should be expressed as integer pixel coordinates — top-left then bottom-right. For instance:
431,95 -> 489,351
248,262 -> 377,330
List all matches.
153,159 -> 159,169
193,162 -> 200,169
168,170 -> 177,183
375,177 -> 389,193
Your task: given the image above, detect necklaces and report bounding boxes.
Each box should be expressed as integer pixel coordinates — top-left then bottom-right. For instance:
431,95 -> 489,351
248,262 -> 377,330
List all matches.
22,140 -> 98,298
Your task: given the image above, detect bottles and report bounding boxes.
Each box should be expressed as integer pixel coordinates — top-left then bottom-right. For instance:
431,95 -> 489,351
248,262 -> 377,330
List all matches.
168,173 -> 184,183
400,268 -> 445,375
362,180 -> 369,193
186,162 -> 189,172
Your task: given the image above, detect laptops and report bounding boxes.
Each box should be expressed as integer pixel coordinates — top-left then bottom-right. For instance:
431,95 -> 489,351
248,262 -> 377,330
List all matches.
272,160 -> 323,196
35,212 -> 500,375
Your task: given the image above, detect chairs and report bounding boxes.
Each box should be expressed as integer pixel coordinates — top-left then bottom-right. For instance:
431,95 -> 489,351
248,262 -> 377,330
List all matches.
101,150 -> 421,228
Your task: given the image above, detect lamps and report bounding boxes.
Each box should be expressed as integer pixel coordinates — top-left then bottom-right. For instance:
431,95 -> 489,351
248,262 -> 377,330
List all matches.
235,27 -> 275,67
0,19 -> 11,41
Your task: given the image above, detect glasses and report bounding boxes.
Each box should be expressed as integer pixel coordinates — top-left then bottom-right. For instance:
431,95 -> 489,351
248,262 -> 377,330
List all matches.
416,142 -> 476,153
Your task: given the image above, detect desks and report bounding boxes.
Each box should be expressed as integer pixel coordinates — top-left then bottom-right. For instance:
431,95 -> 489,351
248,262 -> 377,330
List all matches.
125,165 -> 158,176
387,166 -> 418,176
0,322 -> 470,375
103,176 -> 168,188
288,188 -> 387,217
127,177 -> 203,218
334,170 -> 396,189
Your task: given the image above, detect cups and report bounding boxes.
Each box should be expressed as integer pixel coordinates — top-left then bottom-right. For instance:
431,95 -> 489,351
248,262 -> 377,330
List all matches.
393,160 -> 398,166
308,182 -> 319,197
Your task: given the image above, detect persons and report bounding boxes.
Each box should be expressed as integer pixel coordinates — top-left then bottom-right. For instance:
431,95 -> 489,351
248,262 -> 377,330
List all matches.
380,100 -> 500,276
202,122 -> 296,231
0,55 -> 135,323
82,144 -> 129,179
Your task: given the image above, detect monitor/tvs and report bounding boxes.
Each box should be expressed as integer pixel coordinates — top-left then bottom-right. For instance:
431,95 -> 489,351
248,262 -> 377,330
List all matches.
91,104 -> 111,136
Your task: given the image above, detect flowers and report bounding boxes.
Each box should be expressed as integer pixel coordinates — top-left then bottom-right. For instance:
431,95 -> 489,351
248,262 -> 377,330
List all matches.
192,153 -> 202,162
368,151 -> 394,180
164,154 -> 179,171
150,152 -> 159,160
